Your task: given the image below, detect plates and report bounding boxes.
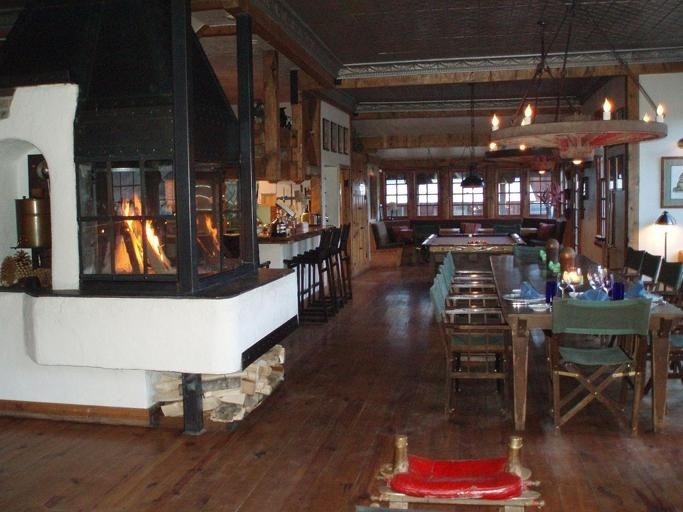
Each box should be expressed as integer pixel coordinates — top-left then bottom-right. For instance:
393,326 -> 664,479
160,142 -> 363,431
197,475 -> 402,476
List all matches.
502,293 -> 546,304
527,303 -> 550,313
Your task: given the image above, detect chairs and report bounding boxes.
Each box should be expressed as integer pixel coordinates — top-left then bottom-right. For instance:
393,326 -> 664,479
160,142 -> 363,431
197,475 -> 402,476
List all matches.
429,250 -> 508,420
282,222 -> 351,327
544,297 -> 652,436
368,220 -> 404,270
622,246 -> 683,398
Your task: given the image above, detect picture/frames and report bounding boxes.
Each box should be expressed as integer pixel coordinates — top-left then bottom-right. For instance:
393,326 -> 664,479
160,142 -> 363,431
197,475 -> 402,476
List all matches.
661,157 -> 683,209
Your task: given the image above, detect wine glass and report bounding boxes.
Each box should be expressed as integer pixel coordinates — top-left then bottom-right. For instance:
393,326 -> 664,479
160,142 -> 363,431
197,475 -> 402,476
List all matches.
558,264 -> 614,302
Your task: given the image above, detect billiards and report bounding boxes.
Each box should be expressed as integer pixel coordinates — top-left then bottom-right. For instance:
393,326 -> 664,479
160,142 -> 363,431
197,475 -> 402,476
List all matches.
467,239 -> 488,244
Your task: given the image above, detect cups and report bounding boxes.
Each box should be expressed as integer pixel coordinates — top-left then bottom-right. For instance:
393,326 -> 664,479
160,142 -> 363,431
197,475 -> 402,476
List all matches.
612,280 -> 624,300
545,280 -> 558,304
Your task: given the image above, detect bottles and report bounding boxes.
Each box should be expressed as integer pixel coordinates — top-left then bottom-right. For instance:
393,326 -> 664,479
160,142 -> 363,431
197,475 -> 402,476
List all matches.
560,239 -> 576,267
545,238 -> 559,261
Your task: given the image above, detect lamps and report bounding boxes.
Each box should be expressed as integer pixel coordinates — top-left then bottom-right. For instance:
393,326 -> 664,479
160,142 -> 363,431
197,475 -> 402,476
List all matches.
654,210 -> 678,261
461,98 -> 484,187
481,3 -> 669,166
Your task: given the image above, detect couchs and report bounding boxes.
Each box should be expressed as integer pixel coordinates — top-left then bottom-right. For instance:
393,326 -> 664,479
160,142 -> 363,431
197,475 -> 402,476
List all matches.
410,219 -> 566,245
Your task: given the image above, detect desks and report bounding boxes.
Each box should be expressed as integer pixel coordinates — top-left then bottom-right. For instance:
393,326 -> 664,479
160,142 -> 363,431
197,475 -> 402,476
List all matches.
488,252 -> 683,435
